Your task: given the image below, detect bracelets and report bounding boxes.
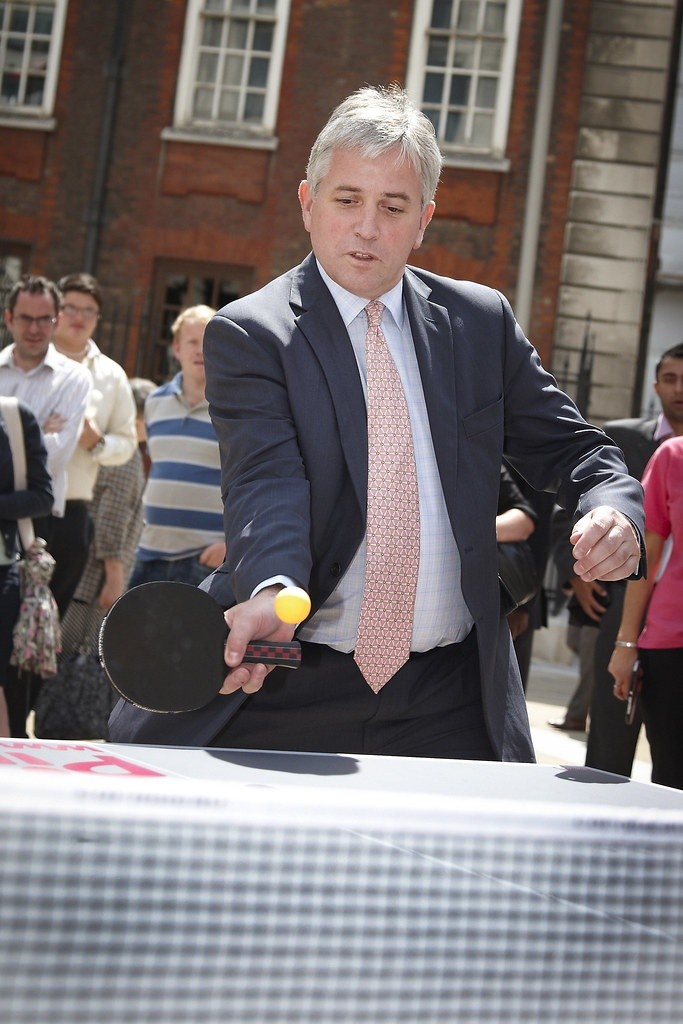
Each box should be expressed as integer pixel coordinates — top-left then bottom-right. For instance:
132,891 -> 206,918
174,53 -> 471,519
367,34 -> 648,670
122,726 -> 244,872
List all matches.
614,640 -> 637,649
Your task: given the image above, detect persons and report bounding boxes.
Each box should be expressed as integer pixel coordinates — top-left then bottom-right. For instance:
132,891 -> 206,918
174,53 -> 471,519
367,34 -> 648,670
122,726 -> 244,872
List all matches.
106,82 -> 649,764
496,345 -> 683,788
0,271 -> 227,739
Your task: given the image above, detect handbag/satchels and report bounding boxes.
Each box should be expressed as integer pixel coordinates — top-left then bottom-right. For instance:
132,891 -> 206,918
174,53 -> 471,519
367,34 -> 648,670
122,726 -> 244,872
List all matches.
6,537 -> 64,679
32,595 -> 120,741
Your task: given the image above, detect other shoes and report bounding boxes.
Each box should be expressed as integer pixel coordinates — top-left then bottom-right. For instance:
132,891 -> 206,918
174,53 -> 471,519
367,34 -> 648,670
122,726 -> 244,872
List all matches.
549,719 -> 586,731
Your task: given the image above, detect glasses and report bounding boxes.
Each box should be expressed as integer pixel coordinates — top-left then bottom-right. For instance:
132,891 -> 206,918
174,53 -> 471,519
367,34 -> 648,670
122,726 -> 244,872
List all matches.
58,304 -> 102,320
9,314 -> 56,326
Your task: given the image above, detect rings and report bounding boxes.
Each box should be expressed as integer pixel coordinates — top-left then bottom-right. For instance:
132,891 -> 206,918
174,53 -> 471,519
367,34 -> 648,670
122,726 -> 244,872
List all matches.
611,684 -> 621,688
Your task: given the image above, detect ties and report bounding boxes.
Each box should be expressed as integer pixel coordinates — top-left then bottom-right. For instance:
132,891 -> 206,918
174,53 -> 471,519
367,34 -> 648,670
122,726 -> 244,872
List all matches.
353,298 -> 419,697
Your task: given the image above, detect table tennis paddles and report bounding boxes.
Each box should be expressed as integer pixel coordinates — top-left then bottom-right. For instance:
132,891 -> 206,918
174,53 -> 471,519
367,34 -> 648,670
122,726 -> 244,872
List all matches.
98,580 -> 301,713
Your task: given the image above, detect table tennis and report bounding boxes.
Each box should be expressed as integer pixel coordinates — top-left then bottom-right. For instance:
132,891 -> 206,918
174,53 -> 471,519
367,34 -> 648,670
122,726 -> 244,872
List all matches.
273,585 -> 311,624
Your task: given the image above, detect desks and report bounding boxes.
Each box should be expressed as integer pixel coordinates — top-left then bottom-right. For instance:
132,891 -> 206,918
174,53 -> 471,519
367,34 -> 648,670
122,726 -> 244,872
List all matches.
0,738 -> 683,1024
